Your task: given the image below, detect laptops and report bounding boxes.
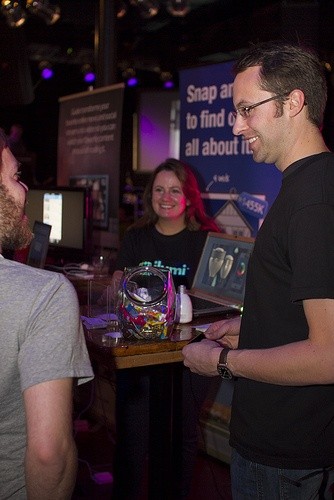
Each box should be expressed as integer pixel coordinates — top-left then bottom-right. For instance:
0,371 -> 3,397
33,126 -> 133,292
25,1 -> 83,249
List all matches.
188,232 -> 255,316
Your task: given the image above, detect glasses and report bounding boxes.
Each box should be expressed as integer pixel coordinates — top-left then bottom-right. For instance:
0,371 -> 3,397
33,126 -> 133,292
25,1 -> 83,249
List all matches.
232,92 -> 308,118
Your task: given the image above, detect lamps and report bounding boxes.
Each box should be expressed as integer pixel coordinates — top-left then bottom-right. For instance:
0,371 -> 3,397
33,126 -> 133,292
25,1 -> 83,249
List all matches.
0,0 -> 61,27
129,0 -> 192,16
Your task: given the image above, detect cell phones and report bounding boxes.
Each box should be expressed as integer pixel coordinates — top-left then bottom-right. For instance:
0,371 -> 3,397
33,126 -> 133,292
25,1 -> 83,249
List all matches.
30,220 -> 52,255
186,333 -> 205,344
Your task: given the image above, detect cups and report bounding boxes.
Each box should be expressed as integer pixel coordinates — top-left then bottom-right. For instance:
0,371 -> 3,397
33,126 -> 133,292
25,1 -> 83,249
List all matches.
106,286 -> 119,326
92,255 -> 110,278
15,245 -> 29,263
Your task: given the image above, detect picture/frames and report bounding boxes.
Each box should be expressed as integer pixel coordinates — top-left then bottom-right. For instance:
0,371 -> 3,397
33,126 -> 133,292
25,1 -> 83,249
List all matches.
69,173 -> 110,229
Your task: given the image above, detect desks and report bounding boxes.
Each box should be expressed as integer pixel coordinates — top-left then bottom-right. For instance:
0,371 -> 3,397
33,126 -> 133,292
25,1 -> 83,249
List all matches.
72,276 -> 241,500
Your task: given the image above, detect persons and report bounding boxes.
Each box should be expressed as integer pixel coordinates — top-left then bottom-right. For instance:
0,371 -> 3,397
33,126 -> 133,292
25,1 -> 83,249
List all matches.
0,128 -> 94,500
98,158 -> 220,500
181,40 -> 334,500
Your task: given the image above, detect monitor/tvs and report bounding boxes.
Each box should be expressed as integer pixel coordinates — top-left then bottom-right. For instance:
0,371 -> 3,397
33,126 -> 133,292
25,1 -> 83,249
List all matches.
22,184 -> 87,252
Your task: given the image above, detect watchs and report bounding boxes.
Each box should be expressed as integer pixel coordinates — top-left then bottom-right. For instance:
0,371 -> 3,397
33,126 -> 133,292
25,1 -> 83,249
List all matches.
217,348 -> 233,379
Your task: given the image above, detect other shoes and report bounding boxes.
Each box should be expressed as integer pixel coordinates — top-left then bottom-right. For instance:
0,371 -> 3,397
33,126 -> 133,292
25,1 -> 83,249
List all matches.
175,472 -> 192,500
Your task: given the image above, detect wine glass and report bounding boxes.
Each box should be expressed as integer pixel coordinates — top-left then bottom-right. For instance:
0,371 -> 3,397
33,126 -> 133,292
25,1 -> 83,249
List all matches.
208,249 -> 234,287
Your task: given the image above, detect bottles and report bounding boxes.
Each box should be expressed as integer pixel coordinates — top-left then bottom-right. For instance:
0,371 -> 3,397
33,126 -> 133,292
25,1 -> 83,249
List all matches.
117,266 -> 181,341
174,285 -> 192,324
